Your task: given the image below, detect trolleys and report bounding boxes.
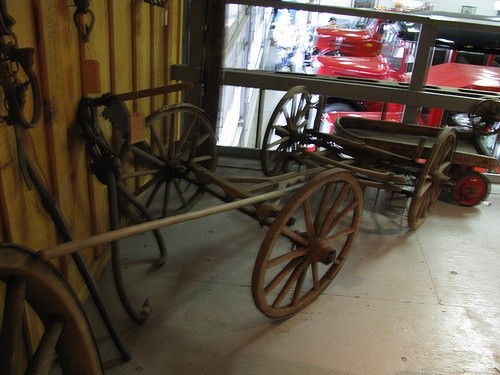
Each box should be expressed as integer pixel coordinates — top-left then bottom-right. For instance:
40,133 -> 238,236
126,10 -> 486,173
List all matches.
34,87 -> 499,318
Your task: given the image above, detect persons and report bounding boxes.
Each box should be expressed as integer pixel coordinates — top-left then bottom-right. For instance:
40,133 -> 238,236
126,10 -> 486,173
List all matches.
268,8 -> 337,73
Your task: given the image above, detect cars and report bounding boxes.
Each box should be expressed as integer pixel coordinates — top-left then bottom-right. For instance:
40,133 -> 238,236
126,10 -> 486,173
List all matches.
309,12 -> 499,151
